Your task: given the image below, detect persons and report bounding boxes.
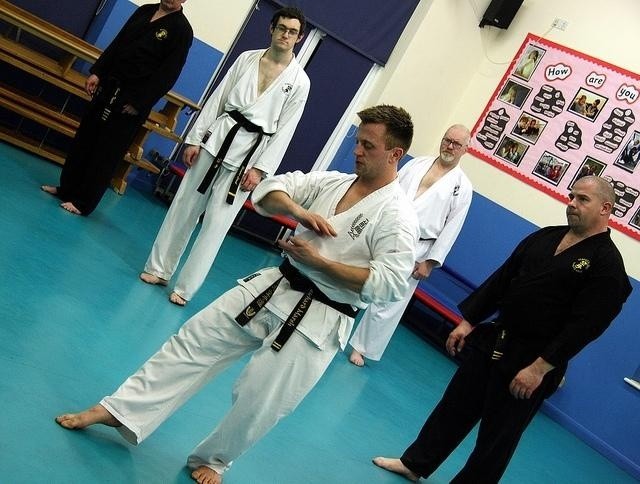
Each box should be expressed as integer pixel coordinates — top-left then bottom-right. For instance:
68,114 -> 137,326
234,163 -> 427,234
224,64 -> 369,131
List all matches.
570,94 -> 586,115
621,139 -> 640,167
546,164 -> 563,181
139,8 -> 313,307
41,0 -> 195,217
506,143 -> 519,162
514,116 -> 529,135
586,98 -> 601,119
52,103 -> 423,484
371,173 -> 634,484
497,140 -> 514,157
504,84 -> 519,104
525,119 -> 538,135
348,122 -> 475,367
577,164 -> 599,179
517,50 -> 540,77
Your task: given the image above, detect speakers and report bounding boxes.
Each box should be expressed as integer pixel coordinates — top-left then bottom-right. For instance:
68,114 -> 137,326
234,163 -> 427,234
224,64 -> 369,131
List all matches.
479,0 -> 524,30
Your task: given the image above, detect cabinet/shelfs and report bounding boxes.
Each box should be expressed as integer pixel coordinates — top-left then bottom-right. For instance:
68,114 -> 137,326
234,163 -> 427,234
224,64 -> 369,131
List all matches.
0,0 -> 201,194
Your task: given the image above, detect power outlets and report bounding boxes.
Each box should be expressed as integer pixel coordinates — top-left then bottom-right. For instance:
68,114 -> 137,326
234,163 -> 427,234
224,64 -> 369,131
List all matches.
551,16 -> 568,30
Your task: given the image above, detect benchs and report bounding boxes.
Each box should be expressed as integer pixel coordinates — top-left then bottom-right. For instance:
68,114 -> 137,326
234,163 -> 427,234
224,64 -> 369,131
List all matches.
166,164 -> 298,247
413,280 -> 461,328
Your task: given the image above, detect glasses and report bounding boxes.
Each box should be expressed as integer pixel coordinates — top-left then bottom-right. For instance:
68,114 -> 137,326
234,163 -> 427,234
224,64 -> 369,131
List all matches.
274,24 -> 298,36
441,136 -> 462,149
